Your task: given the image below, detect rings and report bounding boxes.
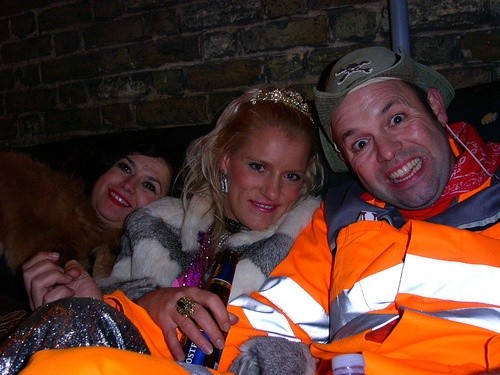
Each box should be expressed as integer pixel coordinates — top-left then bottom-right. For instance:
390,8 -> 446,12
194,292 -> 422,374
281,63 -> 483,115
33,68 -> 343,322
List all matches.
175,296 -> 196,317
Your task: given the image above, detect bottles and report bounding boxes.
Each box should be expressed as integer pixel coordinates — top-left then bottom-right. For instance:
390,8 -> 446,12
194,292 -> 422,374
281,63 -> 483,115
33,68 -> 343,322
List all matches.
330,353 -> 366,375
183,249 -> 240,371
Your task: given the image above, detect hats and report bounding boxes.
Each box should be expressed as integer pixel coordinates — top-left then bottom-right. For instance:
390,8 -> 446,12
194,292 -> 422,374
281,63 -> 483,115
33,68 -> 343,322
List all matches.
312,46 -> 455,173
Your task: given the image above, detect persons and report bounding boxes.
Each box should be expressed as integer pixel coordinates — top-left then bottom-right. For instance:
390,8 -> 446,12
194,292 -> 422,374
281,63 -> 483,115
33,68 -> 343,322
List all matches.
109,84 -> 321,363
0,144 -> 180,313
23,47 -> 500,374
